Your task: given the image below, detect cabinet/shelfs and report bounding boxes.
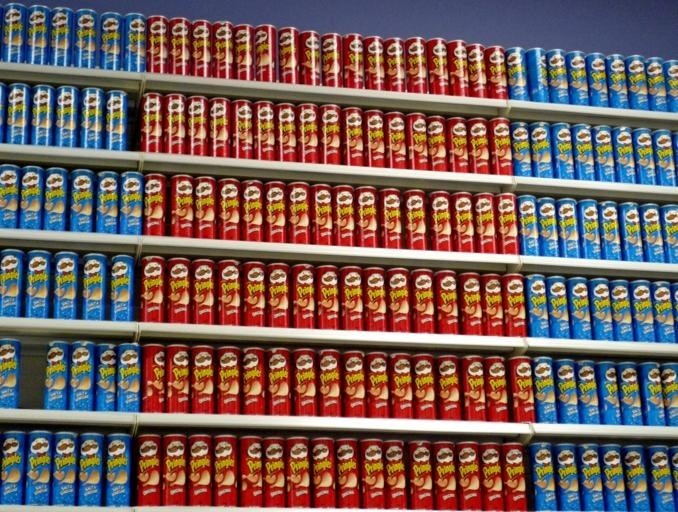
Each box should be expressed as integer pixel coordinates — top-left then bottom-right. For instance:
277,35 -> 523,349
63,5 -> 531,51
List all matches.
1,62 -> 677,512
